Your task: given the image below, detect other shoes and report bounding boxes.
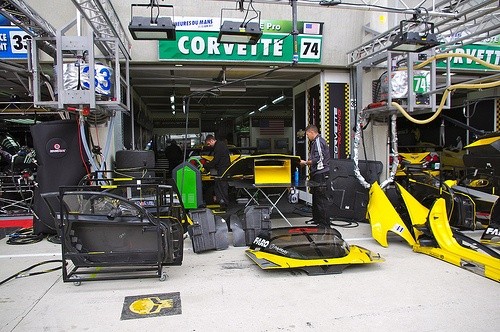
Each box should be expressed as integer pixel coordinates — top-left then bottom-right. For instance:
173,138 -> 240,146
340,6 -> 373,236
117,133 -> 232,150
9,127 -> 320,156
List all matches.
306,219 -> 316,225
215,208 -> 226,211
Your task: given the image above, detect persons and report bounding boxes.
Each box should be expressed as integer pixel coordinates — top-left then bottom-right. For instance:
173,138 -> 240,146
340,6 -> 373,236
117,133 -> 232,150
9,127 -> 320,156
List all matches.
205,134 -> 230,212
298,124 -> 331,226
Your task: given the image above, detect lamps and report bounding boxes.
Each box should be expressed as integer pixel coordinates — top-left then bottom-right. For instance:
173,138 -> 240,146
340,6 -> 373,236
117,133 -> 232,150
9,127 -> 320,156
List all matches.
128,0 -> 176,40
217,0 -> 263,45
387,8 -> 441,53
272,90 -> 287,105
